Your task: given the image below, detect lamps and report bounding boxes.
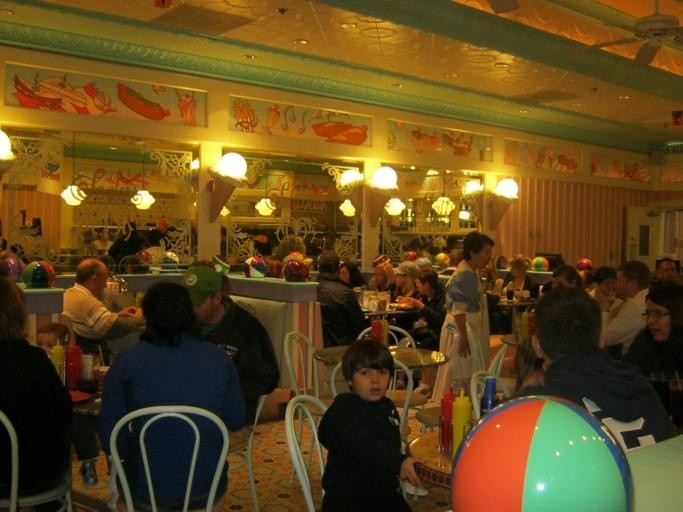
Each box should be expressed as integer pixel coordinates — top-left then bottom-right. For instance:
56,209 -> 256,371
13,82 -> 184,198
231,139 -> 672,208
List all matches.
130,138 -> 156,211
431,169 -> 520,231
60,131 -> 87,207
339,166 -> 399,231
208,151 -> 279,225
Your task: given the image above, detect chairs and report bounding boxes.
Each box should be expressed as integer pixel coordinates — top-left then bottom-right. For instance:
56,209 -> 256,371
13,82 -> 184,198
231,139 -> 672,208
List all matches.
0,371 -> 269,509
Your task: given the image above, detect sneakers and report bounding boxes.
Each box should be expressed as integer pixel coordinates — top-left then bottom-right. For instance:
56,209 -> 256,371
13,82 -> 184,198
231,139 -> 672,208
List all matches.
414,383 -> 431,398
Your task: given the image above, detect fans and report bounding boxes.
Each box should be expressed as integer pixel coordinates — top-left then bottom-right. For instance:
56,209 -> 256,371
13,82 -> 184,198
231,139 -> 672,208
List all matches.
560,0 -> 683,72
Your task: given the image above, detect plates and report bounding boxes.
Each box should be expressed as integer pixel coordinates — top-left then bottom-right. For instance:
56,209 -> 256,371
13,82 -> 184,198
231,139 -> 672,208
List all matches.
499,296 -> 516,302
390,302 -> 415,310
67,391 -> 90,402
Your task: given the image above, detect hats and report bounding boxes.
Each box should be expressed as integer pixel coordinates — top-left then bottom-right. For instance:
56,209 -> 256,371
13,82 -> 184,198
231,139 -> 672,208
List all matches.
178,264 -> 221,304
394,261 -> 421,276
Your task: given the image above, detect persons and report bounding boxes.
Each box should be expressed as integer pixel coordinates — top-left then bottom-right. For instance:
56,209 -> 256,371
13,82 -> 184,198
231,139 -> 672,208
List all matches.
316,339 -> 427,512
0,276 -> 75,511
496,285 -> 683,511
624,281 -> 683,433
1,221 -> 683,421
94,279 -> 249,511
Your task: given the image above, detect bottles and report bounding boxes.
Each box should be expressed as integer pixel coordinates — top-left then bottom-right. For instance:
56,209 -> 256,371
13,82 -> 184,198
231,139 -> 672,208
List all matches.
372,315 -> 390,350
480,377 -> 501,420
51,337 -> 94,391
438,383 -> 471,460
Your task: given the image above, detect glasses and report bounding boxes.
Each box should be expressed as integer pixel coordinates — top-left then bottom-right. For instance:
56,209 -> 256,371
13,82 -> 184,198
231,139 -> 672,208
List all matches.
642,310 -> 671,321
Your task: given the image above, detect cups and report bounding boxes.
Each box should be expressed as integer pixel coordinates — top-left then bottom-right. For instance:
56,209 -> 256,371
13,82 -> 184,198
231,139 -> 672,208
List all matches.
357,289 -> 390,312
96,366 -> 111,394
506,285 -> 514,299
397,343 -> 412,358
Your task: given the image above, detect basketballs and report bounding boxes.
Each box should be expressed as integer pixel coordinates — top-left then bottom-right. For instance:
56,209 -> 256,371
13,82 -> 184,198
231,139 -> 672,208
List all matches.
1,254 -> 23,283
243,257 -> 266,278
577,258 -> 592,271
373,255 -> 389,270
436,253 -> 450,271
533,258 -> 550,272
452,396 -> 634,512
163,252 -> 180,269
25,260 -> 56,288
207,254 -> 230,274
285,260 -> 309,282
137,252 -> 153,265
493,255 -> 509,271
